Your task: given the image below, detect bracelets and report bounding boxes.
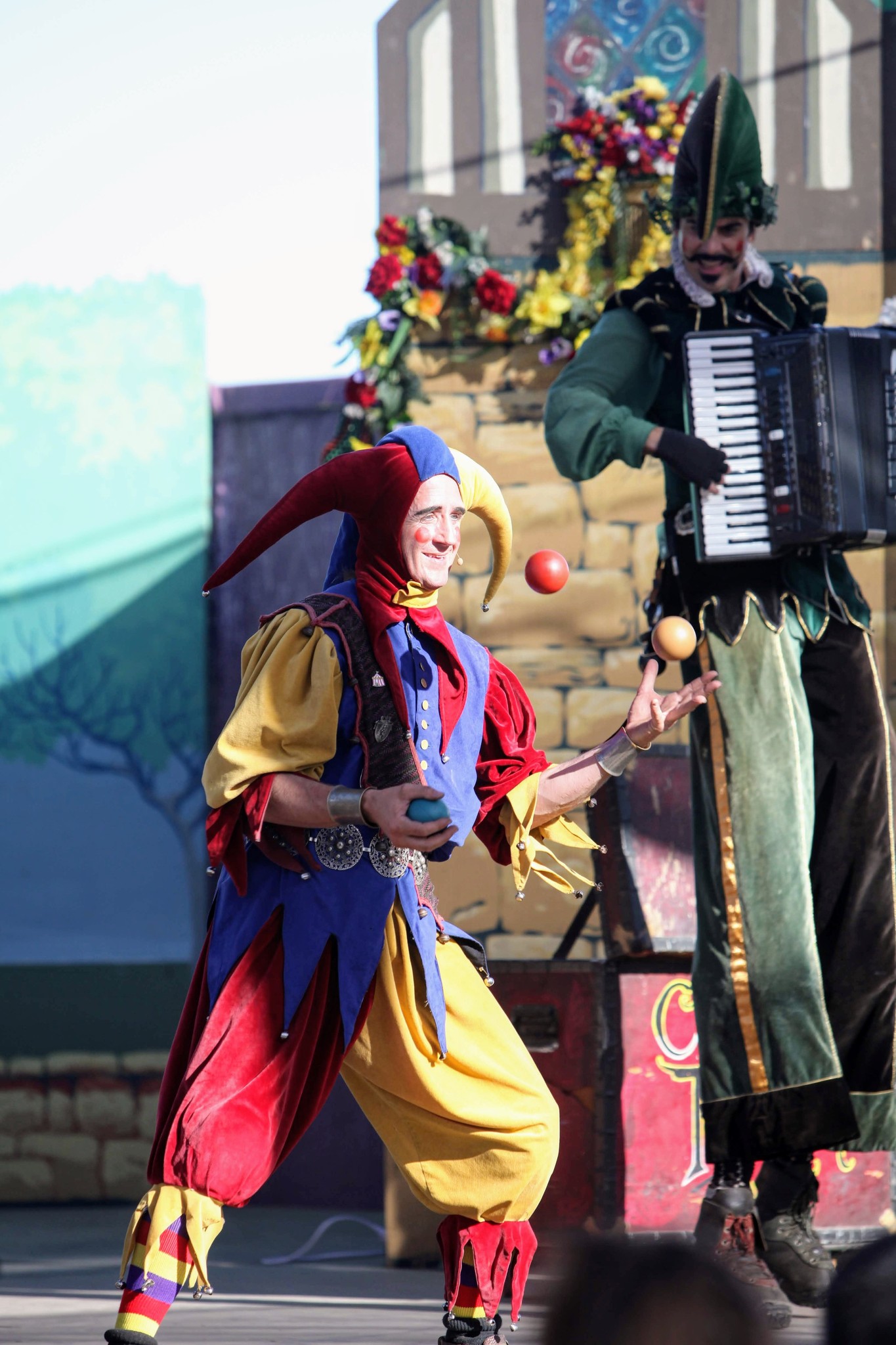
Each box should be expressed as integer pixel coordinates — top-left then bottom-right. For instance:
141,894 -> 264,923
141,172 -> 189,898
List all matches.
594,727 -> 651,777
326,785 -> 378,829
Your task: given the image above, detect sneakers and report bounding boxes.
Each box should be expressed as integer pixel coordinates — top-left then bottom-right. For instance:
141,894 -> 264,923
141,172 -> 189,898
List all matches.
692,1186 -> 792,1328
751,1201 -> 835,1309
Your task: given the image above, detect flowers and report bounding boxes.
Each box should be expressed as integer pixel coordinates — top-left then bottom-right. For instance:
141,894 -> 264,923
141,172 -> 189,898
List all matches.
319,69 -> 698,458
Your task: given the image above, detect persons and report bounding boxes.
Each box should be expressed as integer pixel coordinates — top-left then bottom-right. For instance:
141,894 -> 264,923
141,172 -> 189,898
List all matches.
546,1240 -> 779,1345
541,68 -> 896,1329
103,427 -> 720,1345
825,1234 -> 896,1345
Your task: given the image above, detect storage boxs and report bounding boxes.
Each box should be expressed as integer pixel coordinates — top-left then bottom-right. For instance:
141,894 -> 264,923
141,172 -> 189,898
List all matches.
479,743 -> 892,1257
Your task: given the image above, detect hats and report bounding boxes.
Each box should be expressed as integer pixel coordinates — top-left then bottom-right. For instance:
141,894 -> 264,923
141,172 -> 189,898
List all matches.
201,427 -> 512,613
671,69 -> 777,243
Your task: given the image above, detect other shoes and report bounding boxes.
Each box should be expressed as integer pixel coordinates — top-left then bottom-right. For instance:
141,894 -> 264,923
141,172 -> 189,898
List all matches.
437,1334 -> 509,1345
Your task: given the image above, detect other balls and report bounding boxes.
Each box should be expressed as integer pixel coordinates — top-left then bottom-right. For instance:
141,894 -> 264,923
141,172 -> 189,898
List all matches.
524,549 -> 569,594
406,797 -> 448,823
651,616 -> 697,661
